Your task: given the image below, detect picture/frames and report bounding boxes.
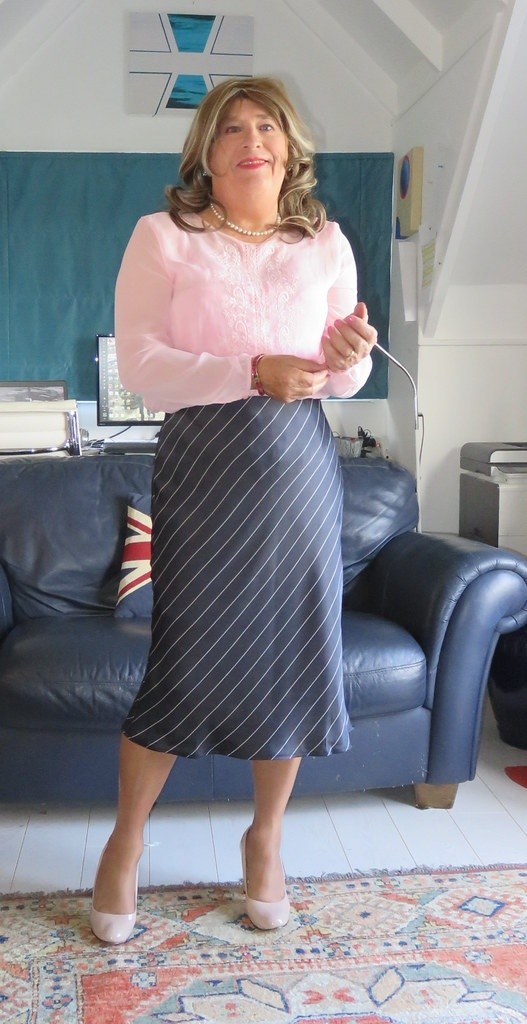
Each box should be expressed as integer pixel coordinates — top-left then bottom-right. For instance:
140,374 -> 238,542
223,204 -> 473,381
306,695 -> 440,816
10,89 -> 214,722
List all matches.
0,381 -> 68,401
96,334 -> 167,425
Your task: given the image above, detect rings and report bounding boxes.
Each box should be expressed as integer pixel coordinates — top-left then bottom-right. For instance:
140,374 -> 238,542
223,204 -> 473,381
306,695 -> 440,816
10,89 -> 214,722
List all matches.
346,350 -> 354,359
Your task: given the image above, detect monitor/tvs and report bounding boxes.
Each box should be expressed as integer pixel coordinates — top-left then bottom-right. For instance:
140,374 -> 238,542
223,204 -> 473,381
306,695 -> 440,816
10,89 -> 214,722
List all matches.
95,334 -> 166,426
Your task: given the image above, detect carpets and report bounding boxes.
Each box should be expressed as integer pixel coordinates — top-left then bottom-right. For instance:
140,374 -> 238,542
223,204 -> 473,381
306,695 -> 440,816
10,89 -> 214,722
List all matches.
2,857 -> 527,1024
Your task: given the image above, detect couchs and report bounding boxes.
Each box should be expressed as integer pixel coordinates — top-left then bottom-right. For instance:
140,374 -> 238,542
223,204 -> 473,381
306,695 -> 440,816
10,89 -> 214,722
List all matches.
2,455 -> 527,809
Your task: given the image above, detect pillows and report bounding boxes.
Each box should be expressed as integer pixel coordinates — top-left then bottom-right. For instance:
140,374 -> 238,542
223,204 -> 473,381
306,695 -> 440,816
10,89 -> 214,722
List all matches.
114,493 -> 152,619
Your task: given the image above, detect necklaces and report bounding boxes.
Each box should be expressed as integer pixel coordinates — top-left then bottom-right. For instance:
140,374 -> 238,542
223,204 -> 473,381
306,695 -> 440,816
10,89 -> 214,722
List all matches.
210,200 -> 281,236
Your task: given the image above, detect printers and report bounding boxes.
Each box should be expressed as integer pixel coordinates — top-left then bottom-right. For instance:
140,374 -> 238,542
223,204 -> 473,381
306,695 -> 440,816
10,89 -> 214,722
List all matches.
458,441 -> 527,556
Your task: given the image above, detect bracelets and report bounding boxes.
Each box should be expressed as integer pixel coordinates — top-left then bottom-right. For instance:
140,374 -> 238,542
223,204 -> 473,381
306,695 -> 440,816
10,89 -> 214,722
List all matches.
252,353 -> 266,395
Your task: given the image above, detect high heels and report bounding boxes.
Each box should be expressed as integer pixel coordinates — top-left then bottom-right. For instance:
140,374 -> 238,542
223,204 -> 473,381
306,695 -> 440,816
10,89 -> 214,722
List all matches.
91,842 -> 139,945
240,827 -> 291,932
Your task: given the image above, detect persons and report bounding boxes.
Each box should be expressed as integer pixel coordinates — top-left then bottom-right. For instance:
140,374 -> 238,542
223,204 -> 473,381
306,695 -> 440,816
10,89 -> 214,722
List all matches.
87,78 -> 379,947
